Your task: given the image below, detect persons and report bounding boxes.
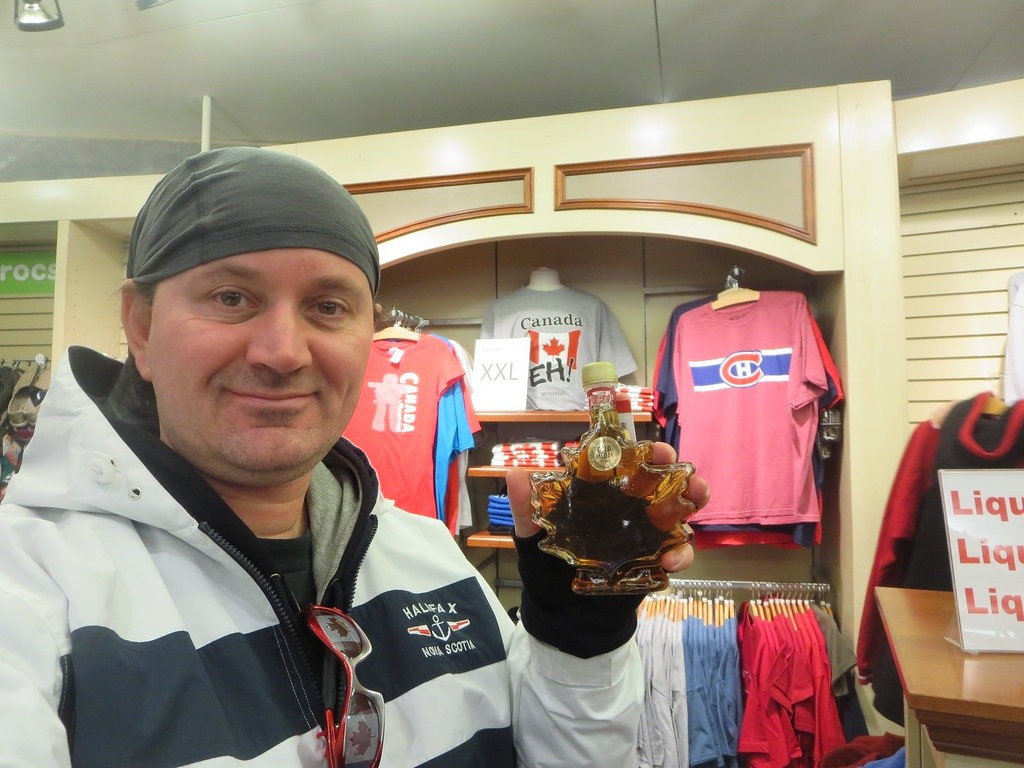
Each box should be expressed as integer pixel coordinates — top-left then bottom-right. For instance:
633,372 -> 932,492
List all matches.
0,147 -> 709,768
482,269 -> 638,411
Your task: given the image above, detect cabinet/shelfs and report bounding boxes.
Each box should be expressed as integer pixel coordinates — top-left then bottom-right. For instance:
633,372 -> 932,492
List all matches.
466,410 -> 653,549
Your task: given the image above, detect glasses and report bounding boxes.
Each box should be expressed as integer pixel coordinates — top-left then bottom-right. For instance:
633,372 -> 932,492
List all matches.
306,606 -> 385,768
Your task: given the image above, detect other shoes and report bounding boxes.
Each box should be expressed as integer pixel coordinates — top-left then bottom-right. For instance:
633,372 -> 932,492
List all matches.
0,362 -> 51,428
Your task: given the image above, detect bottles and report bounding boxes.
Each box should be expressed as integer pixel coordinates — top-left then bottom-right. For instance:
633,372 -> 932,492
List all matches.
530,362 -> 695,594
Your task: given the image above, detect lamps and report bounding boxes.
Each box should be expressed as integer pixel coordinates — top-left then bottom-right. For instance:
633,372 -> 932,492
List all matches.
12,0 -> 65,32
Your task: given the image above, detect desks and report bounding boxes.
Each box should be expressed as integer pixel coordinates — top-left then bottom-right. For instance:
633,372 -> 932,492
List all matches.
874,586 -> 1024,768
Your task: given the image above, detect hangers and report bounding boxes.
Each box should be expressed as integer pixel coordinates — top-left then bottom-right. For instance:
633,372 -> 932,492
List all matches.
637,577 -> 834,631
372,306 -> 424,345
711,268 -> 760,311
